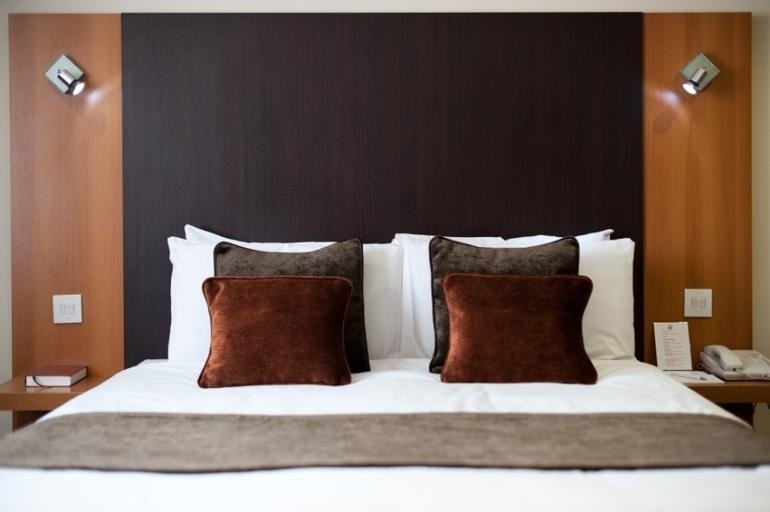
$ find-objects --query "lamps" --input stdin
[678,52,720,97]
[43,53,87,99]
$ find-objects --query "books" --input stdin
[25,363,88,387]
[665,367,724,385]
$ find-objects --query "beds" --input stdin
[1,355,770,512]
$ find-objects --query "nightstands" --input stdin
[662,367,770,405]
[0,373,110,412]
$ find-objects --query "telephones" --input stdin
[699,344,770,380]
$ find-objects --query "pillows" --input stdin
[198,276,353,388]
[440,273,601,384]
[397,228,614,354]
[213,238,372,374]
[165,237,378,361]
[424,237,636,361]
[427,235,581,373]
[181,224,401,357]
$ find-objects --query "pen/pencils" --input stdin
[679,375,706,380]
